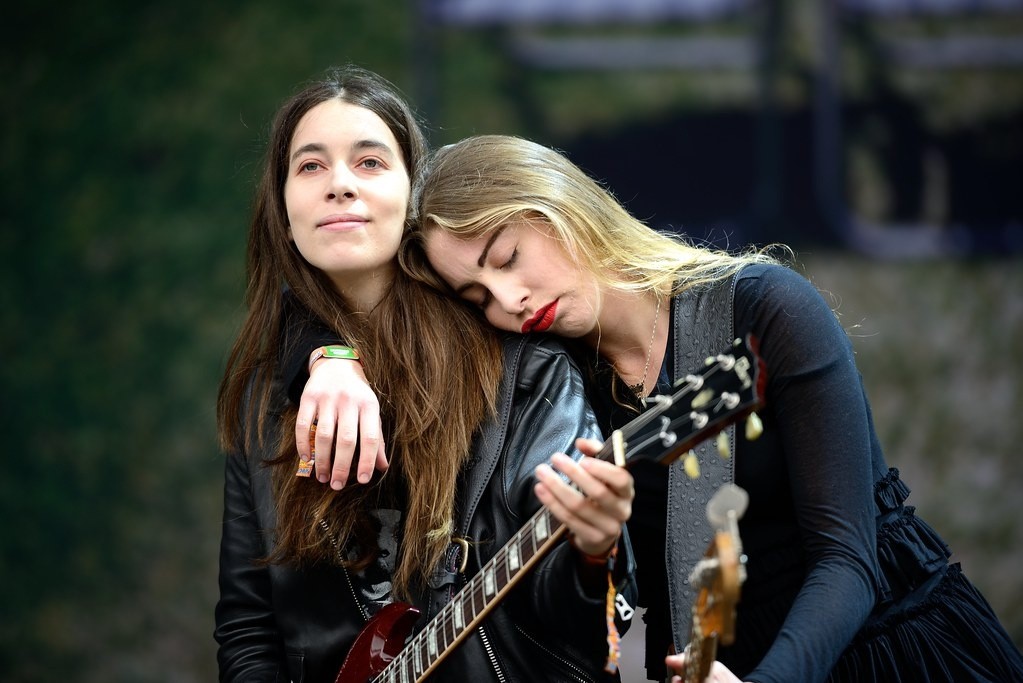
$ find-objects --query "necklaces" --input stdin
[606,296,663,395]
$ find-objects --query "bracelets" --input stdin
[308,345,363,371]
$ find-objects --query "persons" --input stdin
[398,133,1021,683]
[215,66,1022,683]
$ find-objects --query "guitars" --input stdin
[678,480,751,683]
[329,326,768,683]
